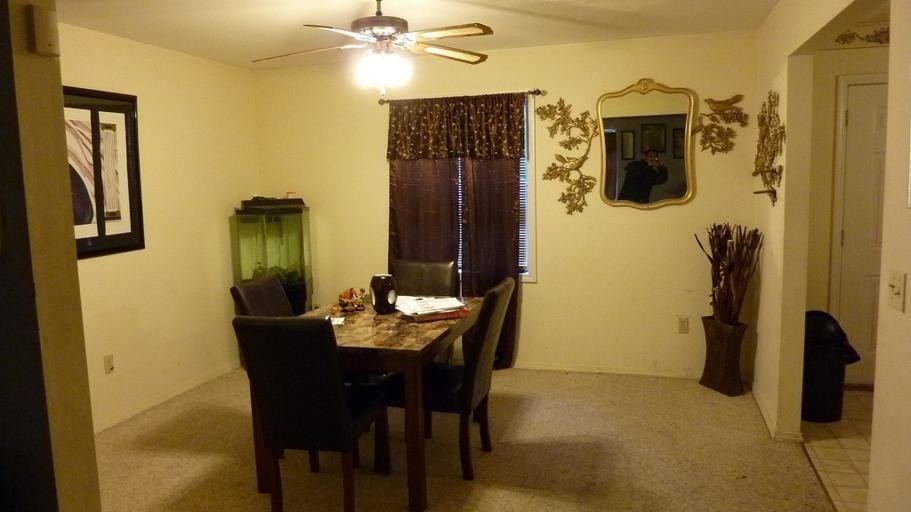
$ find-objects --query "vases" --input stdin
[699,315,748,397]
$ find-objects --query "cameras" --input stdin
[652,154,660,161]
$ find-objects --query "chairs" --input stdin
[229,272,307,459]
[391,259,460,296]
[372,276,516,480]
[231,314,390,512]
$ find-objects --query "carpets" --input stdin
[94,366,835,511]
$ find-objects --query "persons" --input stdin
[617,149,668,203]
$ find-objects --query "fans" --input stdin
[249,0,492,65]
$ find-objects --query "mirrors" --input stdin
[596,78,698,211]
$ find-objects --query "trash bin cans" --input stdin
[802,310,861,423]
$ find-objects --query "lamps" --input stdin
[354,50,414,94]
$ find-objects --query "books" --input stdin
[394,295,470,322]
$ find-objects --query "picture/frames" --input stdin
[620,130,634,160]
[61,84,146,261]
[641,122,666,154]
[673,128,684,159]
